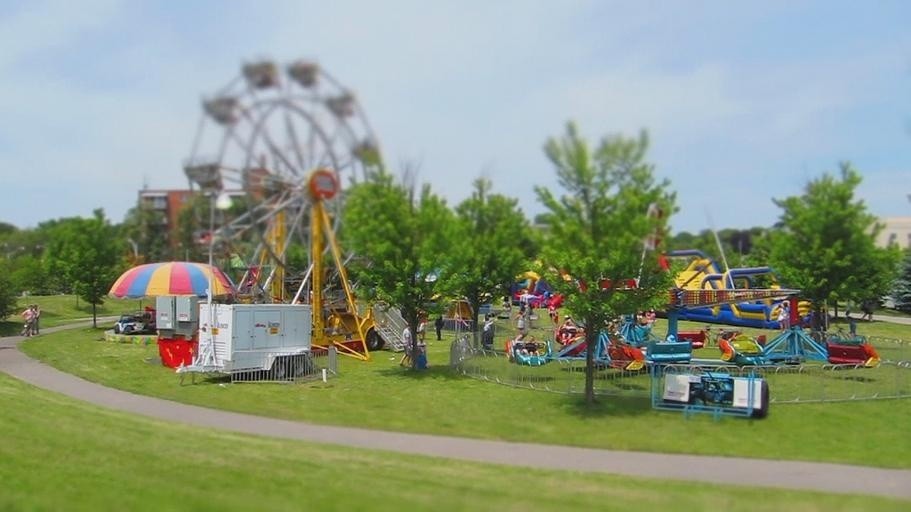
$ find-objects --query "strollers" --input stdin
[21,317,37,336]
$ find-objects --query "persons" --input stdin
[400,295,855,369]
[31,304,40,336]
[21,305,37,335]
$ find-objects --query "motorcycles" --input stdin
[114,314,154,335]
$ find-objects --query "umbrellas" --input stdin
[108,262,238,342]
